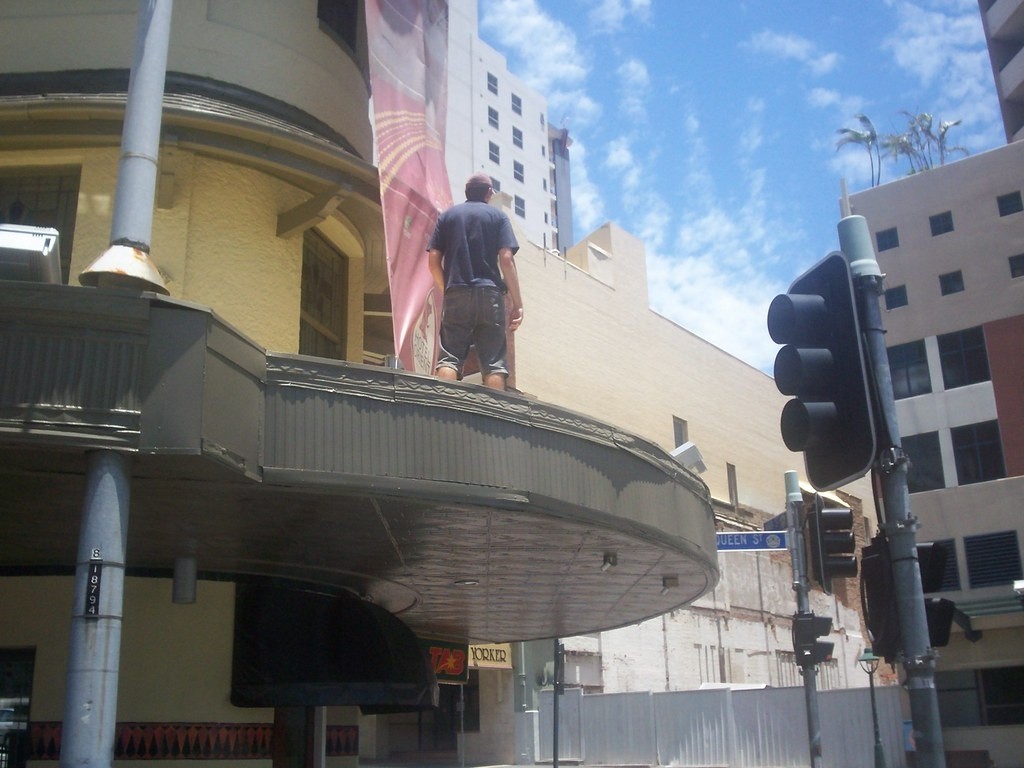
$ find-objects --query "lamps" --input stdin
[601,552,618,571]
[172,555,196,605]
[661,576,680,597]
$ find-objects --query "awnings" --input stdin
[230,575,441,716]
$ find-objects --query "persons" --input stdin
[427,173,524,393]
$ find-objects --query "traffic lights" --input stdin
[915,539,947,576]
[926,599,956,648]
[809,503,858,594]
[810,609,835,665]
[768,250,878,490]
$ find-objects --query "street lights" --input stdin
[858,646,890,768]
